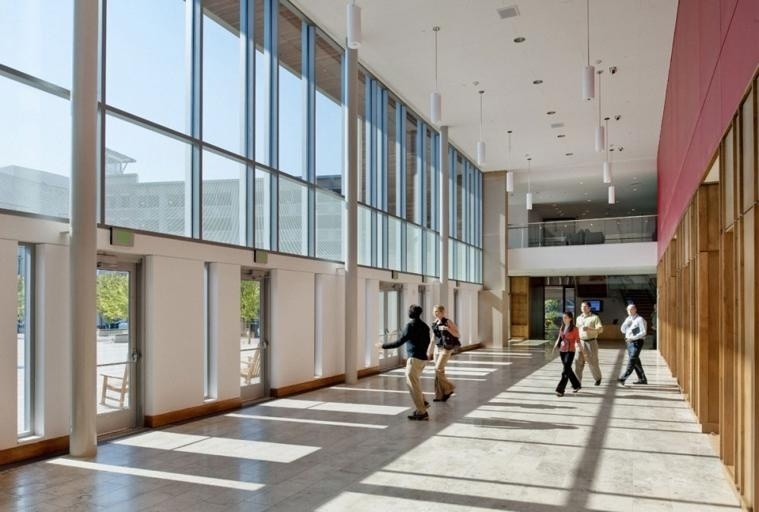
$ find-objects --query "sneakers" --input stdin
[407,401,431,421]
[554,390,565,396]
[572,385,581,393]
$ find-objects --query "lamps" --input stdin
[506,131,514,193]
[526,158,533,210]
[594,70,604,153]
[604,117,611,184]
[346,0,363,49]
[609,149,615,205]
[477,90,486,167]
[582,1,594,101]
[431,27,442,125]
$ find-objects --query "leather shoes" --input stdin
[433,391,454,402]
[617,378,647,386]
[594,378,601,386]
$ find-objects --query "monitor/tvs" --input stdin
[583,300,603,313]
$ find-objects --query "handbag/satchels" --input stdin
[632,327,644,347]
[441,319,461,350]
[557,336,562,348]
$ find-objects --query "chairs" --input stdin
[100,364,127,407]
[240,343,261,385]
[376,334,395,358]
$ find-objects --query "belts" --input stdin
[583,338,597,342]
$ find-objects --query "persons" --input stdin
[615,303,649,386]
[427,303,461,403]
[372,303,431,421]
[572,300,604,391]
[649,303,657,350]
[551,309,592,397]
